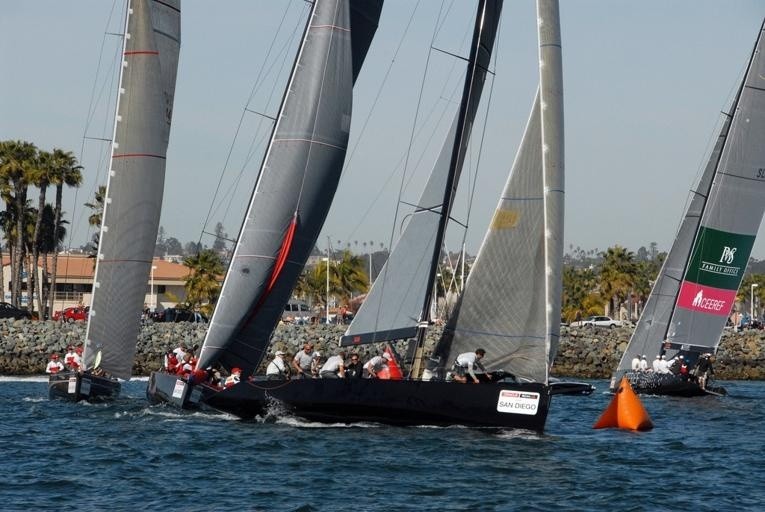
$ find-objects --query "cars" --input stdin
[582,316,622,329]
[0,302,32,320]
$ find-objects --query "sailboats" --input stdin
[609,17,765,396]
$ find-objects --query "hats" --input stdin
[275,351,286,356]
[382,352,393,362]
[47,344,83,359]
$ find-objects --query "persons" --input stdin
[287,302,347,326]
[576,309,583,328]
[163,341,256,391]
[422,368,438,381]
[45,340,84,374]
[727,315,765,333]
[142,301,204,323]
[631,352,716,390]
[266,342,404,380]
[446,349,491,384]
